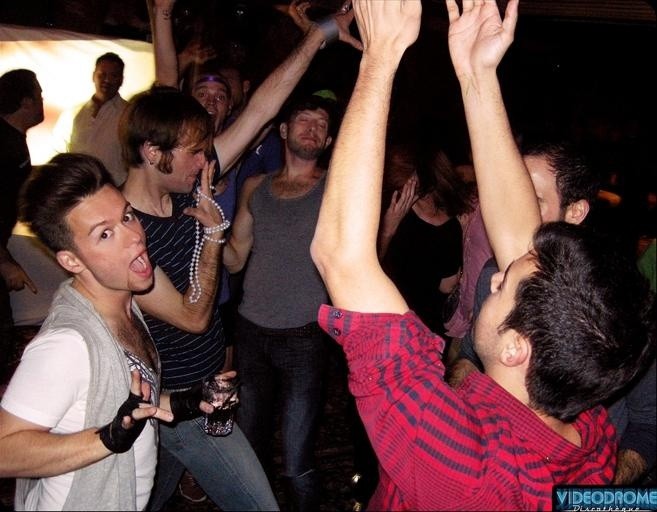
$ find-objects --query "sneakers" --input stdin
[178,470,206,503]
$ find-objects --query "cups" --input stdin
[201,374,242,438]
